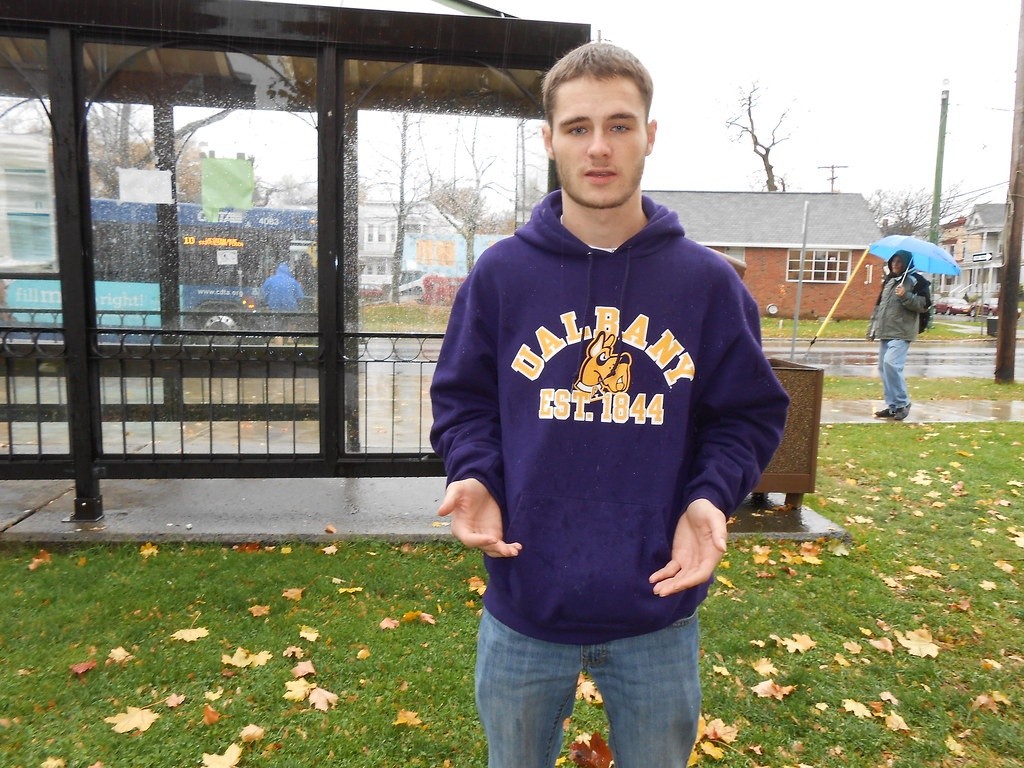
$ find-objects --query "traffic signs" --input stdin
[972,252,992,262]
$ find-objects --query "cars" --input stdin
[934,296,971,315]
[970,298,1021,320]
[357,284,382,297]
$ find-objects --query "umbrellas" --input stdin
[866,234,962,287]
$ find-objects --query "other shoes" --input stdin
[893,402,911,420]
[875,409,896,417]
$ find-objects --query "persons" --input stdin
[427,41,795,768]
[258,262,305,310]
[297,252,319,294]
[865,250,930,421]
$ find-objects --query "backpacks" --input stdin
[910,273,932,334]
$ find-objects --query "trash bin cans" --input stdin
[750,358,825,508]
[986,315,998,335]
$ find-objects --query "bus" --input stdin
[0,193,317,345]
[388,231,515,307]
